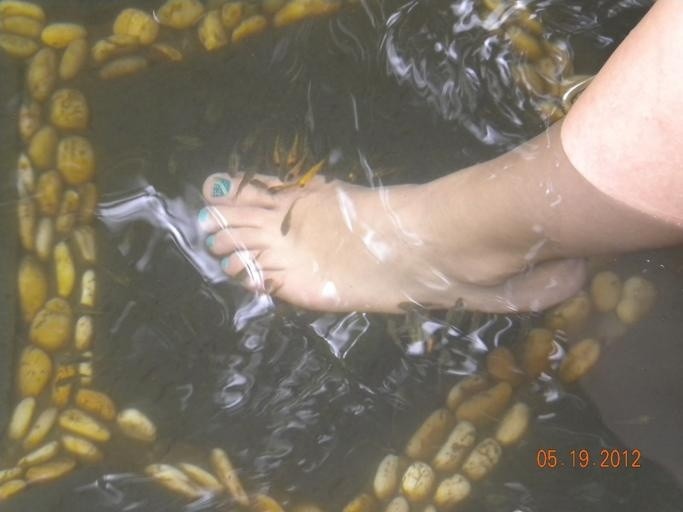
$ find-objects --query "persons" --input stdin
[197,1,682,315]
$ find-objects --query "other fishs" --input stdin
[57,0,682,511]
